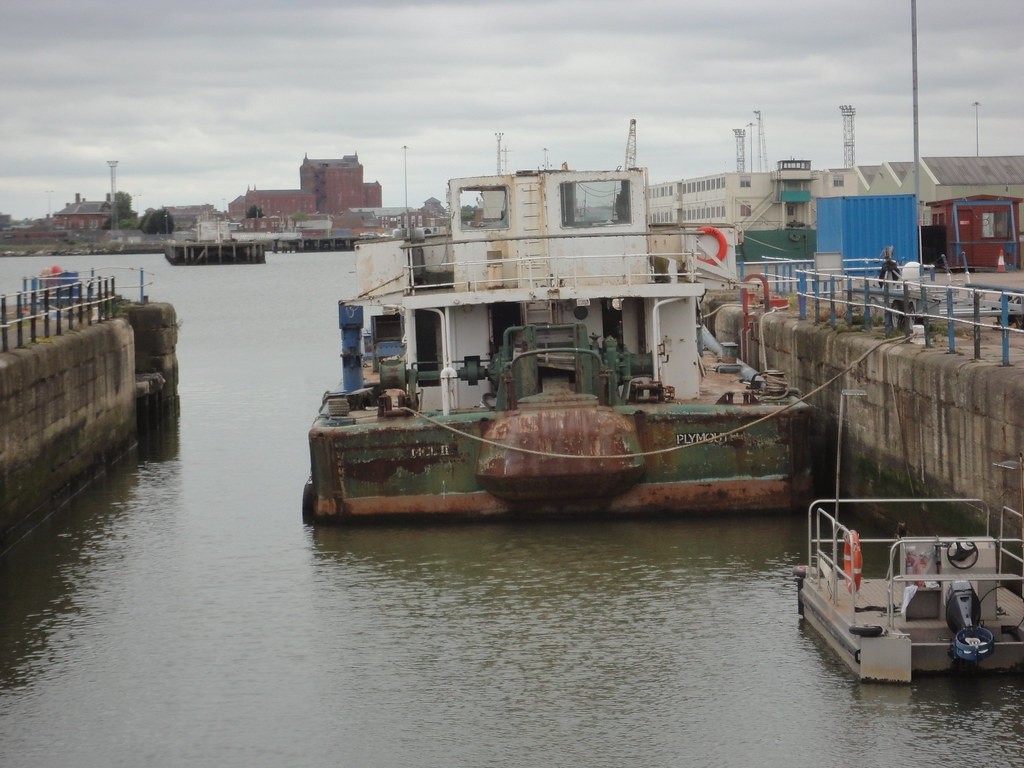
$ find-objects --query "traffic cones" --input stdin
[997,250,1007,274]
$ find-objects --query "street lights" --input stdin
[399,146,411,227]
[971,102,982,156]
[747,123,758,173]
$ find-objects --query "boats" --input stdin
[794,497,1024,683]
[302,165,820,526]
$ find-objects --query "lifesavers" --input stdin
[693,226,728,266]
[843,530,863,593]
